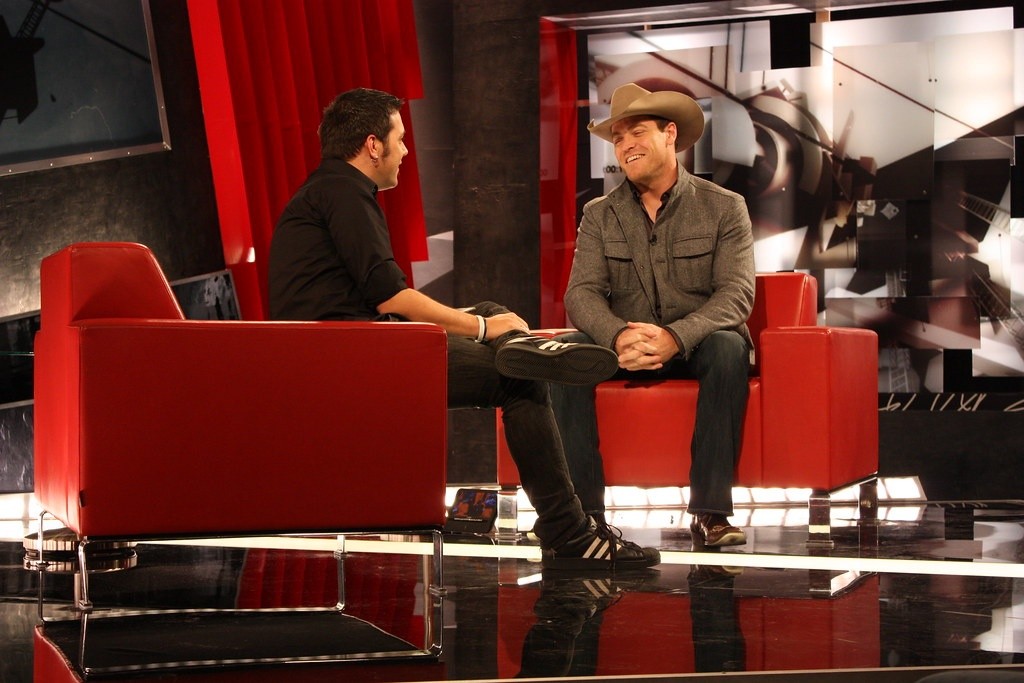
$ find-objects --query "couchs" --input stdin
[32,241,448,675]
[496,273,878,600]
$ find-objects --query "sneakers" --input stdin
[537,567,663,619]
[527,528,541,542]
[494,335,620,389]
[539,517,662,570]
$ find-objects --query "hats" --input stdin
[585,84,706,153]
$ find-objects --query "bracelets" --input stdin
[475,315,487,343]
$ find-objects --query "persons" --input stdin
[546,82,756,546]
[267,88,662,570]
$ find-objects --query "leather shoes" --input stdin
[689,563,746,585]
[686,513,750,548]
